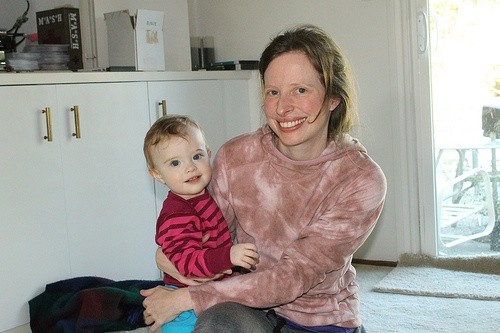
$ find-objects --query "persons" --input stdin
[143,112,259,332]
[140,24,387,333]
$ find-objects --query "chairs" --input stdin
[435,140,496,247]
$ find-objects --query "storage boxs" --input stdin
[37,8,83,72]
[104,7,165,71]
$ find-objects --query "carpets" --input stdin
[373,252,500,300]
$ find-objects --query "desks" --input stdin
[445,143,500,251]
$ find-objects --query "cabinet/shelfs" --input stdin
[0,70,266,332]
